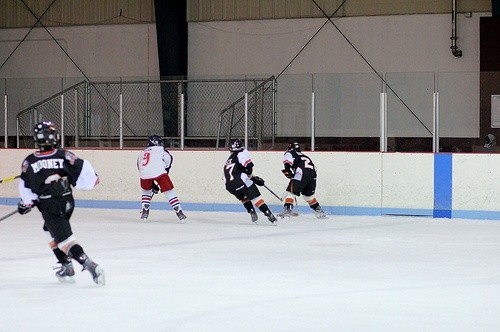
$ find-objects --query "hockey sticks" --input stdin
[290,177,298,208]
[140,184,159,213]
[0,208,18,222]
[263,184,289,201]
[0,173,22,184]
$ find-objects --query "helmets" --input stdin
[148,134,161,146]
[32,121,57,145]
[288,140,301,152]
[230,138,244,151]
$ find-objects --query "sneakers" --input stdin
[264,209,277,225]
[251,211,258,225]
[140,208,149,221]
[81,258,105,286]
[176,210,188,223]
[283,204,293,220]
[56,261,76,281]
[315,206,329,219]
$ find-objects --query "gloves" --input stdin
[251,176,264,186]
[152,184,159,194]
[282,170,294,178]
[18,201,31,214]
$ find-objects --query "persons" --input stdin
[137,135,189,223]
[276,141,329,220]
[17,121,105,284]
[223,138,279,225]
